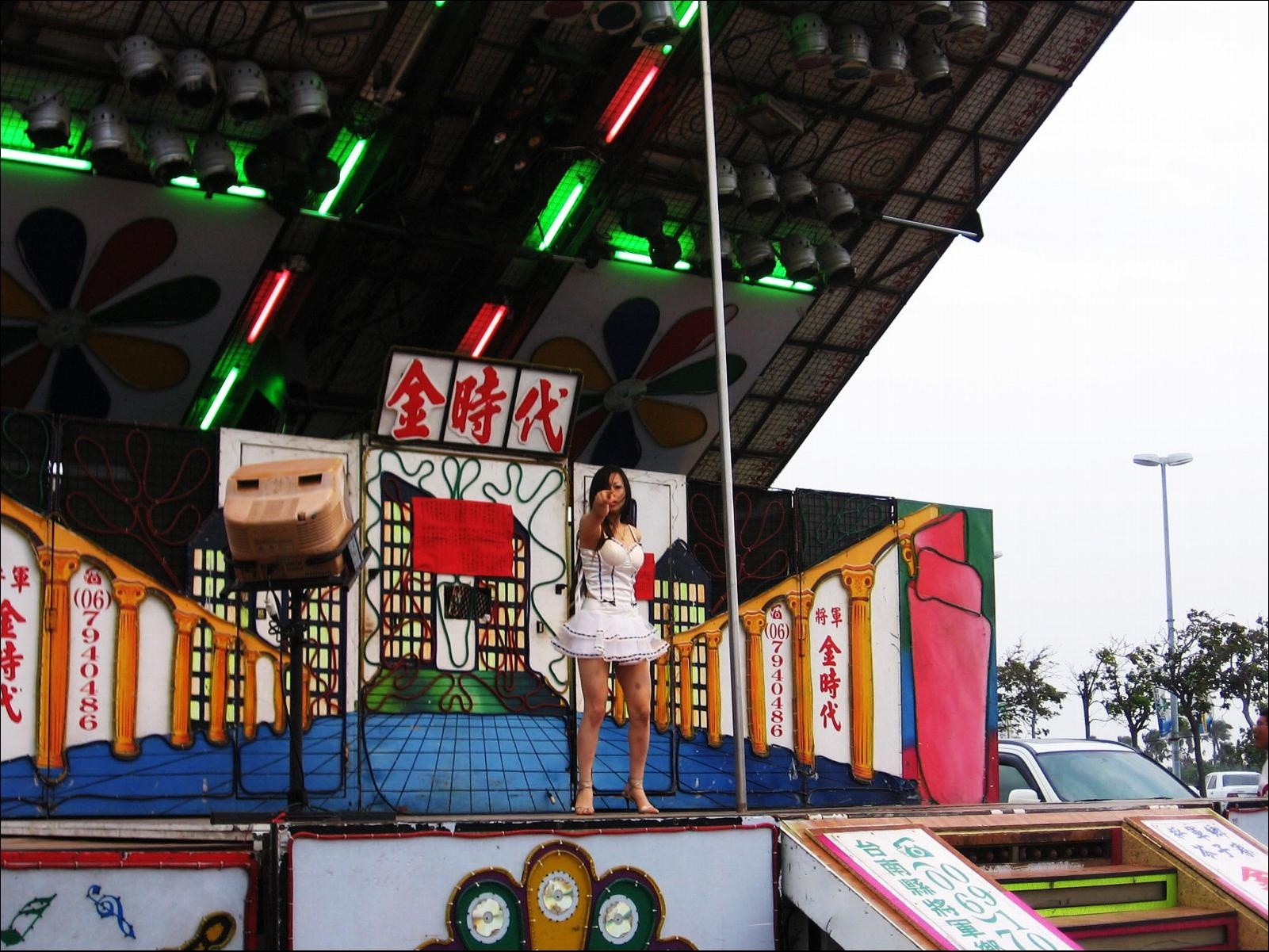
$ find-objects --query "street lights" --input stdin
[1132,452,1193,783]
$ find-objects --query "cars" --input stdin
[990,738,1210,813]
[1204,771,1264,815]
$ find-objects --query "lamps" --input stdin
[695,228,732,276]
[779,171,817,216]
[199,363,241,431]
[739,90,807,144]
[703,158,742,209]
[292,0,391,40]
[170,47,218,105]
[22,86,72,150]
[538,178,584,250]
[738,165,780,211]
[86,98,131,165]
[530,0,685,47]
[195,130,237,189]
[144,119,193,182]
[285,70,331,127]
[781,233,821,282]
[819,183,856,217]
[734,232,777,280]
[117,33,170,99]
[648,231,681,270]
[225,62,273,122]
[788,0,989,94]
[814,239,858,286]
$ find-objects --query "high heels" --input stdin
[622,778,659,815]
[575,781,595,815]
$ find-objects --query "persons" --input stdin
[551,466,671,815]
[1252,706,1269,797]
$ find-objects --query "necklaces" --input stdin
[617,525,626,541]
